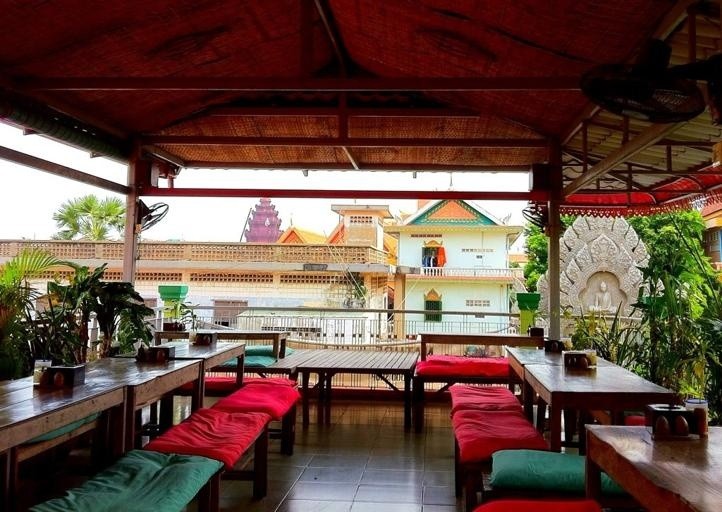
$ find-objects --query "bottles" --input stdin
[38,371,65,388]
[568,357,588,369]
[654,415,688,436]
[138,344,144,362]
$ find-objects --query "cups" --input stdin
[687,398,711,435]
[34,361,52,382]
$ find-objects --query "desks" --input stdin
[296,348,419,433]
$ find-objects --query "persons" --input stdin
[586,281,619,316]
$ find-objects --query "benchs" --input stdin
[416,334,722,511]
[0,330,301,512]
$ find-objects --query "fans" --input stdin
[133,199,170,232]
